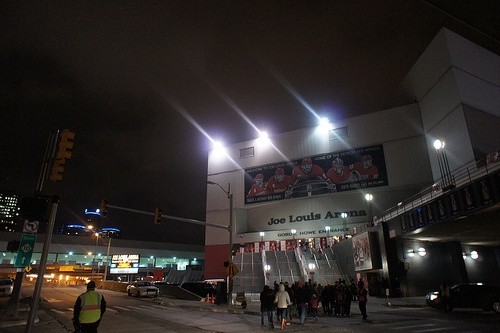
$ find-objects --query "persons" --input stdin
[305,239,338,260]
[373,277,400,298]
[260,277,367,329]
[248,174,268,196]
[440,280,452,316]
[292,158,327,183]
[71,281,106,333]
[265,168,295,192]
[10,276,14,281]
[204,281,215,286]
[348,155,378,178]
[339,236,348,241]
[266,271,272,279]
[326,158,351,183]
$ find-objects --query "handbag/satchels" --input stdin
[358,296,365,301]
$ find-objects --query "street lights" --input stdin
[207,179,236,313]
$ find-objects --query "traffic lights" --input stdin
[50,159,67,184]
[100,199,108,216]
[56,128,77,160]
[154,207,161,225]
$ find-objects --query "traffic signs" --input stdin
[14,233,36,270]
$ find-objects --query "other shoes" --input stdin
[362,318,366,320]
[261,323,264,325]
[315,318,318,320]
[270,326,274,328]
[301,323,304,327]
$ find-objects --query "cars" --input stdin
[0,279,16,296]
[126,280,160,297]
[425,283,500,314]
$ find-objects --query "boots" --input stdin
[280,319,287,330]
